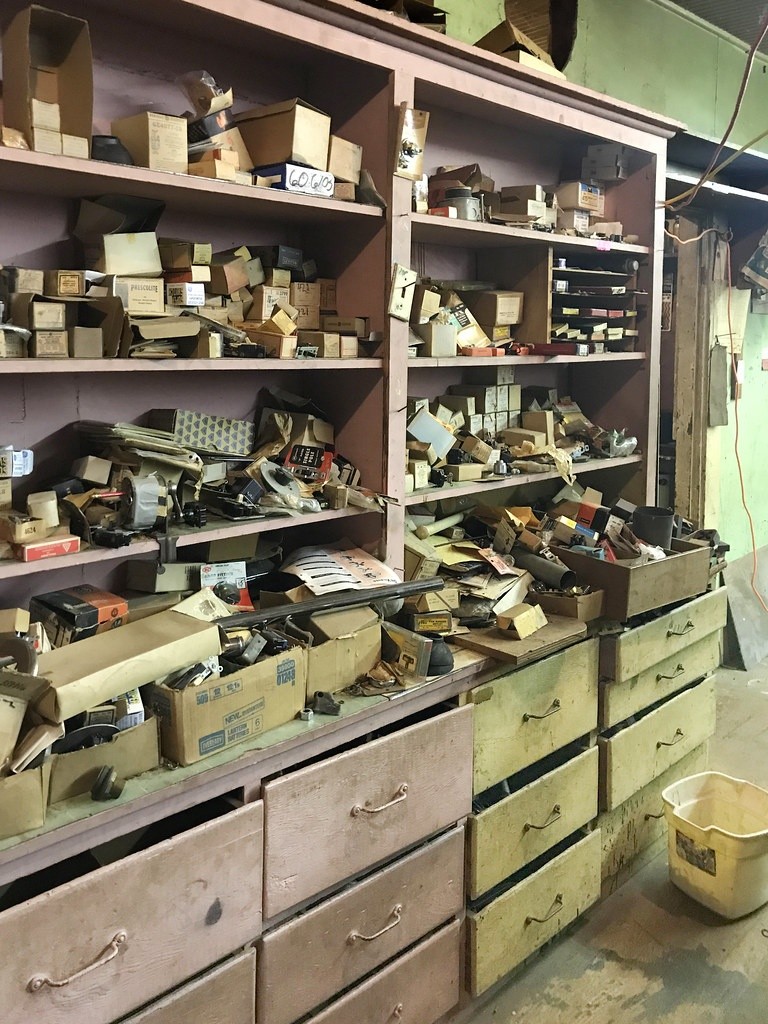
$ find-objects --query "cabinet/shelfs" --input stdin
[2,0,686,608]
[459,584,726,996]
[2,586,474,1023]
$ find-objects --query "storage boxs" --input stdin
[661,771,768,920]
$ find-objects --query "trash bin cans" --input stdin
[659,771,768,920]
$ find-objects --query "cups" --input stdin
[632,506,674,551]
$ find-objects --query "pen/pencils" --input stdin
[88,492,125,498]
